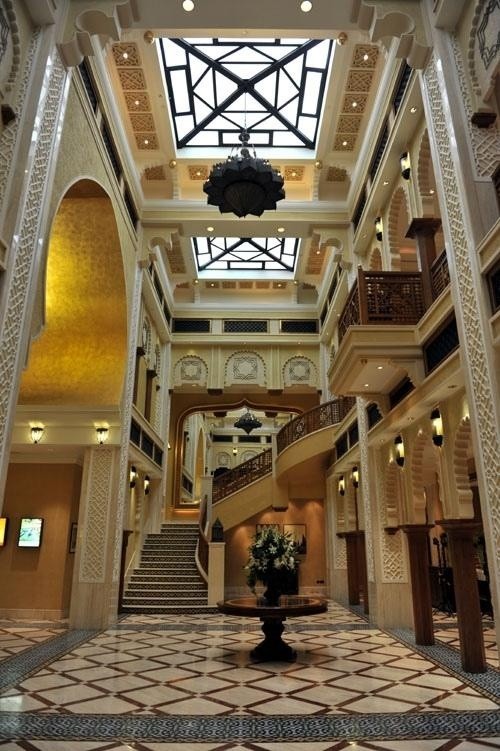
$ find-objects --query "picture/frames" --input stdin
[68,523,79,554]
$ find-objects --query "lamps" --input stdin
[203,80,288,222]
[233,409,262,437]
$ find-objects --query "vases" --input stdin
[262,578,281,602]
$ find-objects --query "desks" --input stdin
[217,596,329,665]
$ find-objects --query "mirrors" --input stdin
[165,384,321,522]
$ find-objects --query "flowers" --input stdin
[241,528,302,598]
